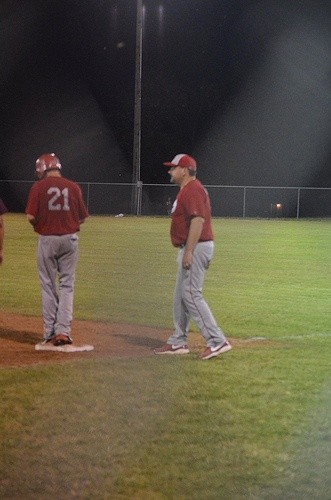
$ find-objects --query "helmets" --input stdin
[35,153,61,177]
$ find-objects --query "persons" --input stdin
[0,199,9,264]
[154,153,232,359]
[25,153,88,346]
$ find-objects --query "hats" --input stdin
[163,154,197,170]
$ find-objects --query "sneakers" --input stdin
[155,344,189,355]
[55,334,71,346]
[35,337,57,348]
[203,341,231,359]
